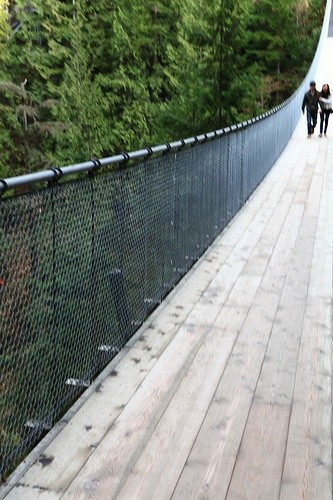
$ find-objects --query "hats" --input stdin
[309,81,316,85]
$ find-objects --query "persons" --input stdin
[302,81,325,139]
[318,84,332,138]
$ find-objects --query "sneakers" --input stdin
[307,134,311,139]
[324,134,328,137]
[317,132,322,137]
[311,125,314,133]
